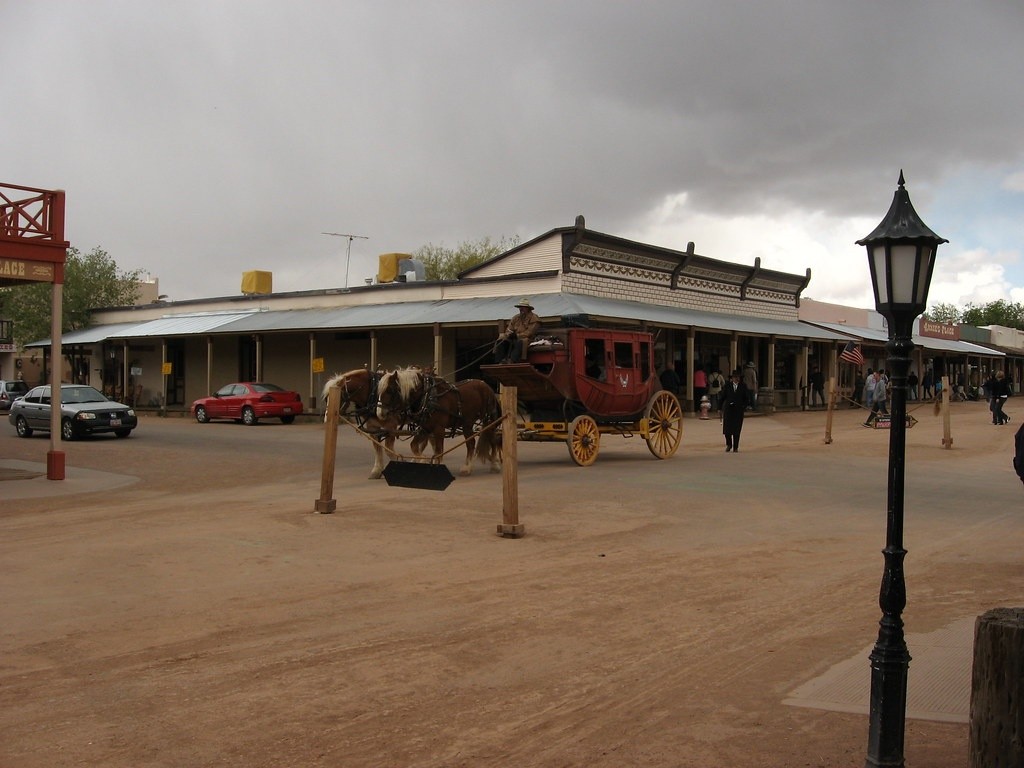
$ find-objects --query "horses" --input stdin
[375,368,502,475]
[322,369,429,479]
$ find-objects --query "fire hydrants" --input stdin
[699,395,712,420]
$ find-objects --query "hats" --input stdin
[728,370,745,379]
[514,298,535,310]
[747,362,757,368]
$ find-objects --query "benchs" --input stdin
[508,337,563,365]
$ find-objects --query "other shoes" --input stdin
[726,444,732,451]
[733,448,738,452]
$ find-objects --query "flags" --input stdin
[840,340,863,365]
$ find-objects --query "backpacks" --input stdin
[712,374,720,388]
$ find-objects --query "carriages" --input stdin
[320,327,684,480]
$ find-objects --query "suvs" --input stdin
[0,379,32,411]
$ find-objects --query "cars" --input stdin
[190,381,304,426]
[8,384,138,442]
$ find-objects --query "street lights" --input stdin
[853,167,952,768]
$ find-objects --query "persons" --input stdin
[1013,422,1024,484]
[921,372,933,401]
[980,369,1011,425]
[799,367,825,407]
[16,369,23,380]
[694,362,758,452]
[494,299,541,363]
[908,372,918,400]
[950,383,964,402]
[659,362,681,418]
[850,368,889,428]
[584,354,601,379]
